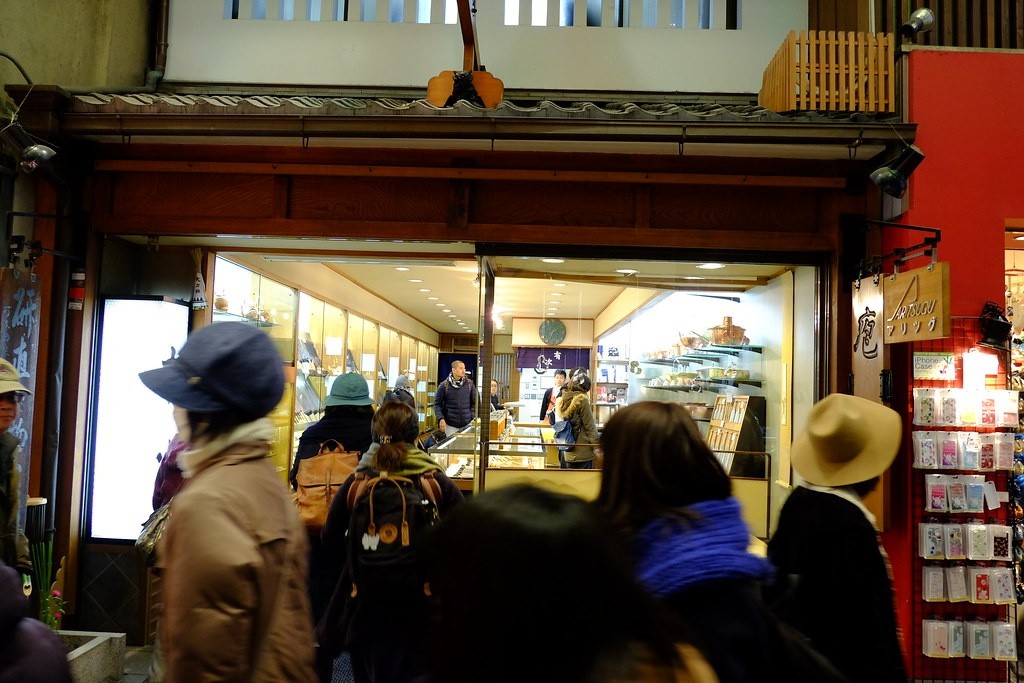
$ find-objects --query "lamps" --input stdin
[902,8,936,45]
[869,144,925,200]
[0,117,58,182]
[975,300,1012,353]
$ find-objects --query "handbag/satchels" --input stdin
[134,503,173,562]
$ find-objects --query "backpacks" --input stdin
[552,413,584,452]
[345,469,443,599]
[293,439,361,534]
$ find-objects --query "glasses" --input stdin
[0,392,24,403]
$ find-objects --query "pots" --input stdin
[649,316,750,387]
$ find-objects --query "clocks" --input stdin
[539,319,566,346]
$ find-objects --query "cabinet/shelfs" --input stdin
[211,251,439,496]
[639,343,763,423]
[590,358,629,432]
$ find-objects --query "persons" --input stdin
[291,371,375,683]
[436,361,480,437]
[408,484,715,683]
[394,374,414,408]
[550,367,602,469]
[539,370,567,419]
[0,357,77,683]
[586,400,774,683]
[323,402,464,683]
[766,390,911,683]
[491,380,504,411]
[139,321,315,683]
[143,433,194,683]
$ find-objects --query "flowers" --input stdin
[39,589,68,633]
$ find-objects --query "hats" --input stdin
[0,358,31,395]
[395,376,410,387]
[789,392,903,487]
[138,320,284,423]
[324,371,375,407]
